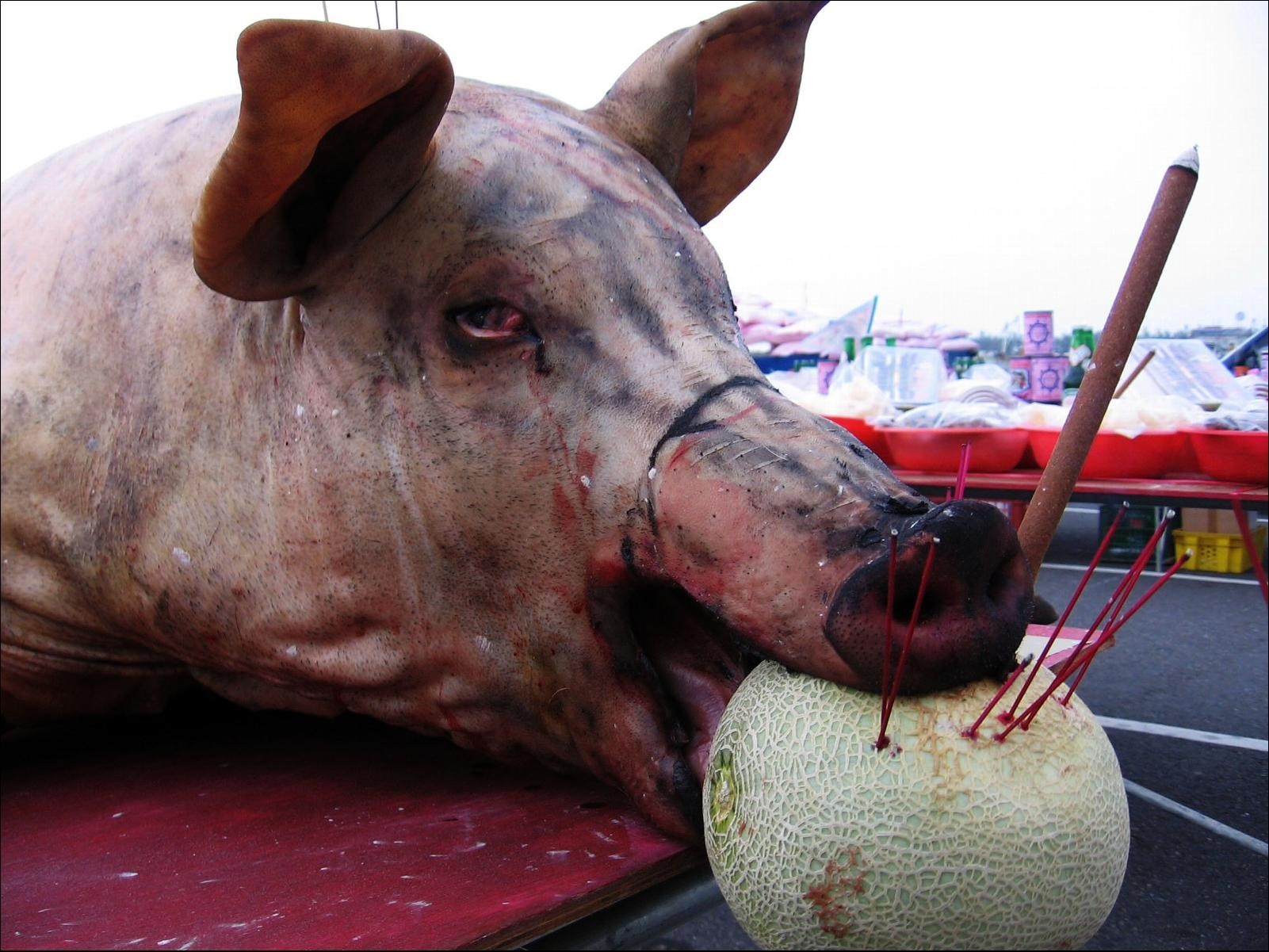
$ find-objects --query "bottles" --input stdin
[1061,326,1097,405]
[843,334,896,366]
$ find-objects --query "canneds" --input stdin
[1007,310,1064,405]
[952,355,982,374]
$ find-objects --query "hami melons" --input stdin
[702,649,1130,951]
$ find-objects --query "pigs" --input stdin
[1,0,1025,848]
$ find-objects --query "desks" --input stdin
[0,625,1115,952]
[891,459,1269,615]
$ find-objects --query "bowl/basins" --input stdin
[820,415,1268,481]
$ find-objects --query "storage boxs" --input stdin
[1182,506,1258,535]
[1047,503,1120,555]
[1172,527,1266,573]
